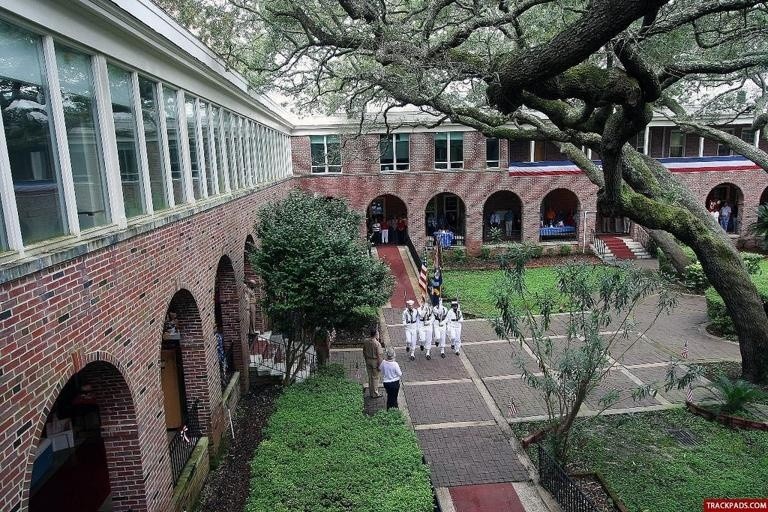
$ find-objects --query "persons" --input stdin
[433,297,448,358]
[707,195,737,234]
[448,301,463,356]
[621,214,631,236]
[212,321,224,377]
[415,296,436,358]
[544,207,556,226]
[427,214,464,249]
[401,298,418,360]
[599,211,613,234]
[363,329,384,400]
[556,209,566,226]
[315,324,330,367]
[504,206,514,237]
[367,214,407,244]
[378,347,404,412]
[490,207,502,238]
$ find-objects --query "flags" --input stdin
[418,248,428,292]
[431,241,442,306]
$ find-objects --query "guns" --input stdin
[404,290,409,324]
[456,288,460,320]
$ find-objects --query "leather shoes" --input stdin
[403,340,463,360]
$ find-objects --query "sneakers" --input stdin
[369,393,383,398]
